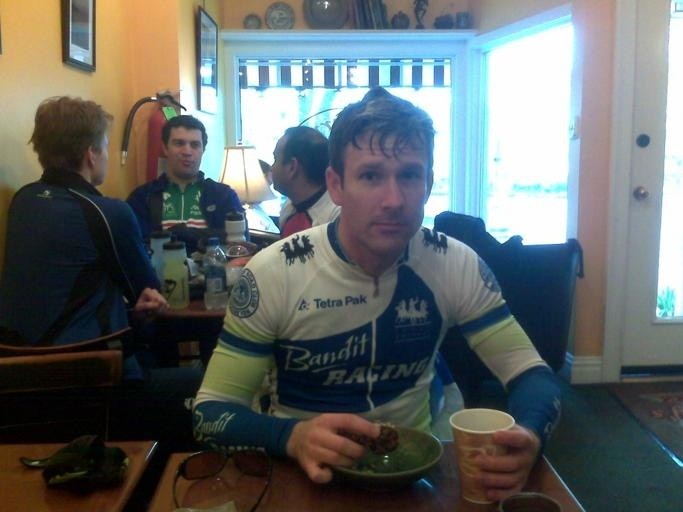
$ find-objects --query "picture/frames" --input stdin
[57,0,100,74]
[193,5,220,118]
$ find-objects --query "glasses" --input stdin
[172,443,274,512]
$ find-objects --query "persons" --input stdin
[2,93,197,439]
[268,125,341,240]
[190,85,562,499]
[243,158,280,235]
[124,113,250,249]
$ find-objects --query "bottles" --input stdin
[149,205,253,312]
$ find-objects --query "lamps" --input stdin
[213,144,277,231]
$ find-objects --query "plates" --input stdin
[303,0,354,30]
[265,2,295,31]
[243,15,261,28]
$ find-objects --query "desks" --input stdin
[0,437,159,509]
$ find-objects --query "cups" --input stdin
[453,12,473,30]
[499,492,565,512]
[448,408,516,506]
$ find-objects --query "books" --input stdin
[348,1,388,30]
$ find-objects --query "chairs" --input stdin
[448,237,582,416]
[0,319,135,381]
[0,346,125,439]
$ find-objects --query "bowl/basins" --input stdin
[323,423,444,489]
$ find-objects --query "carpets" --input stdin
[539,384,683,510]
[600,378,682,471]
